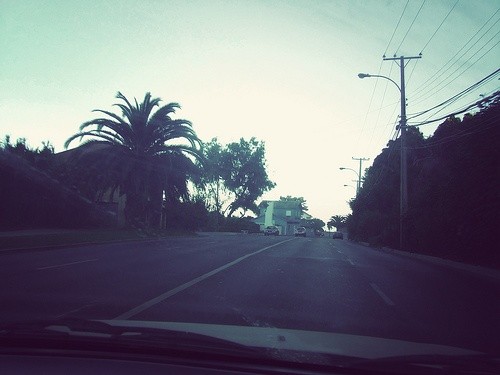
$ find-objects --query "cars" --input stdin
[332,231,344,240]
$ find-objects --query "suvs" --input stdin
[294,226,307,236]
[263,226,279,236]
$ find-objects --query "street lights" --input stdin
[339,156,371,198]
[357,55,424,251]
